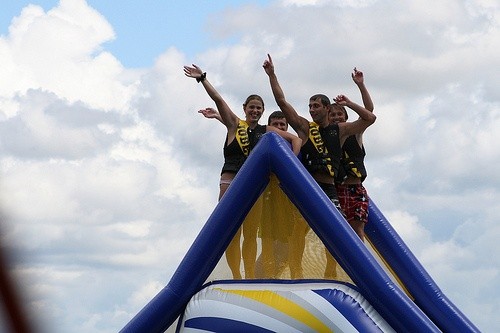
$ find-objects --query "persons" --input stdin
[262,52,377,279]
[198,108,293,279]
[183,63,304,280]
[328,68,374,244]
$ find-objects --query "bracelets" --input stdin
[196,72,207,83]
